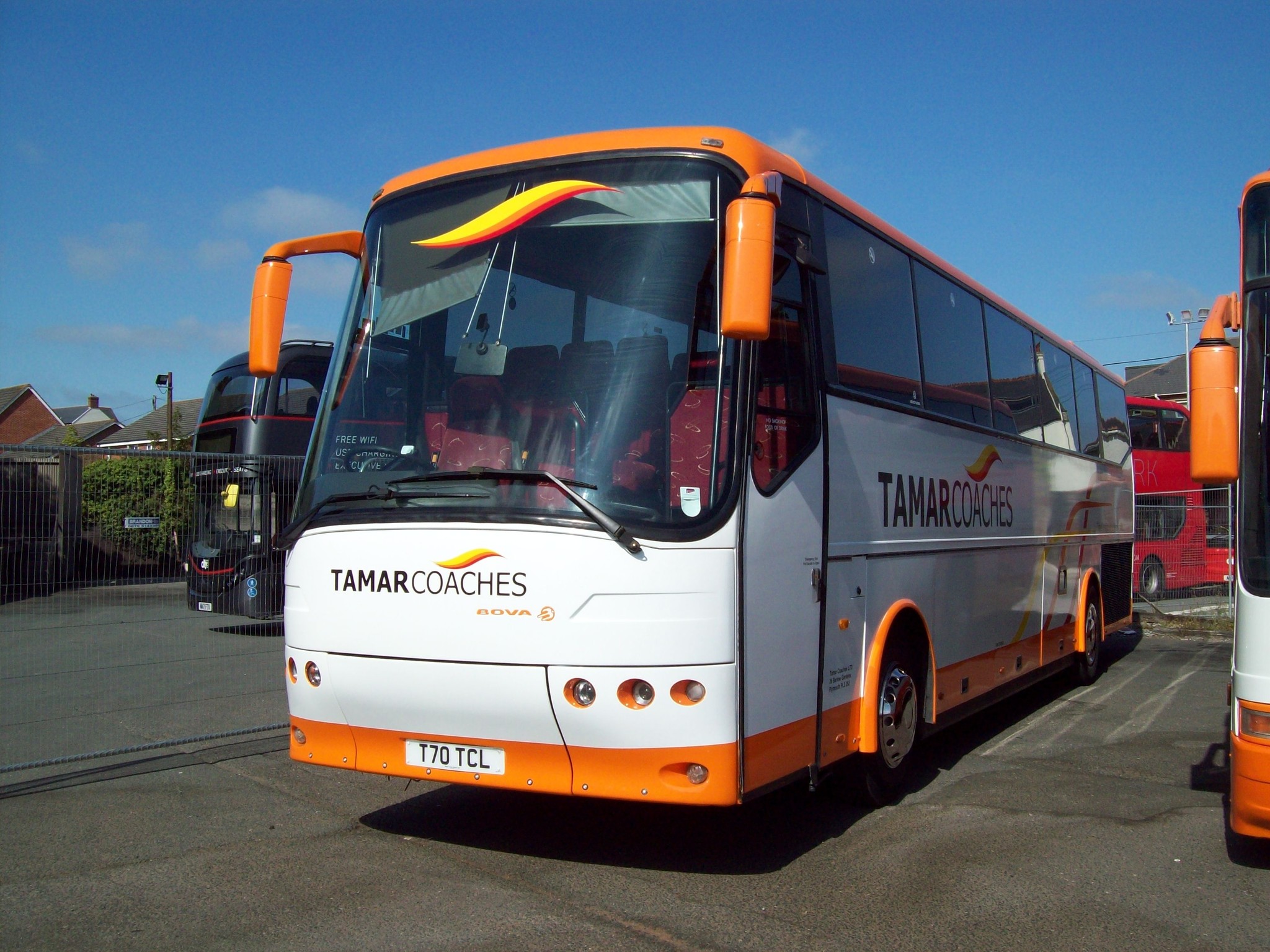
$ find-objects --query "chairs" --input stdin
[421,335,672,509]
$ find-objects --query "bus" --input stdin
[1123,395,1236,602]
[249,125,1138,818]
[185,337,339,620]
[1192,168,1270,840]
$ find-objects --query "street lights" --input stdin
[157,372,174,451]
